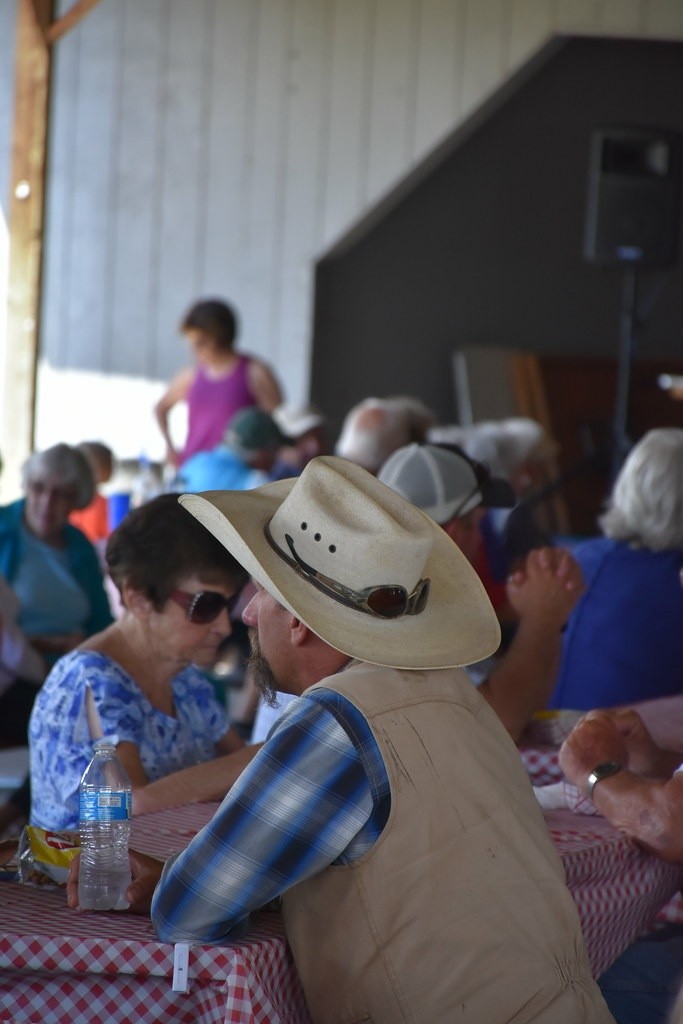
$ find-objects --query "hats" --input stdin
[177,456,502,671]
[377,443,484,523]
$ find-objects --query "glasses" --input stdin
[284,536,432,620]
[149,582,239,623]
[440,443,488,525]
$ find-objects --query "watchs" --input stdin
[587,760,621,800]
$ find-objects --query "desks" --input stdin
[0,747,683,1024]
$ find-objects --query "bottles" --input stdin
[77,741,133,910]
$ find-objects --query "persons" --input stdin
[547,429,683,711]
[0,441,161,750]
[178,394,586,750]
[558,705,683,861]
[24,491,275,833]
[150,297,284,467]
[69,456,617,1024]
